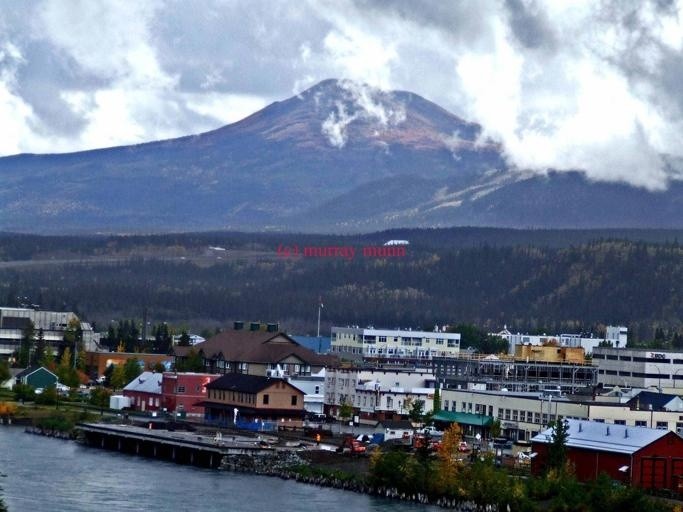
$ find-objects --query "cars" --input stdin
[373,425,519,456]
